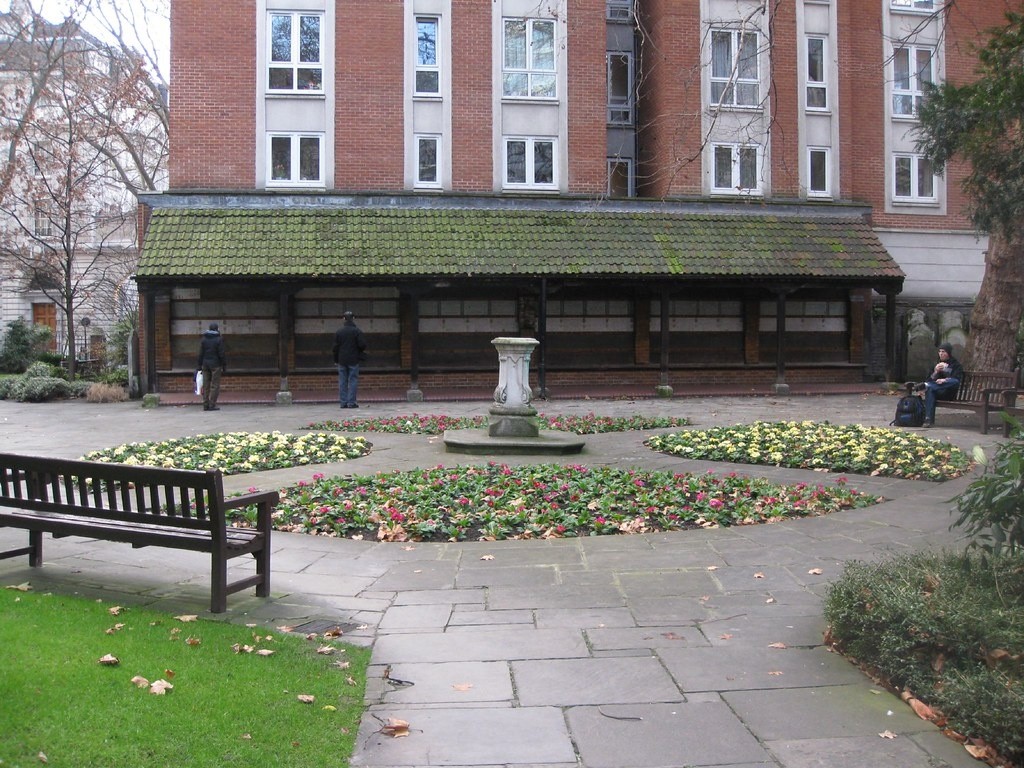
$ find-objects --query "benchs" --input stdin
[0,452,280,613]
[59,359,100,384]
[903,368,1020,434]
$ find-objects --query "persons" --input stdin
[332,311,366,408]
[197,323,226,411]
[912,343,963,428]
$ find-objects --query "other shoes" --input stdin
[204,407,220,411]
[349,404,359,408]
[914,382,925,392]
[340,404,348,408]
[922,421,935,428]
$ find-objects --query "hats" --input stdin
[939,343,952,353]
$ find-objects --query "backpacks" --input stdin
[890,395,924,427]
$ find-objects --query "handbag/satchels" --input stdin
[194,371,204,396]
